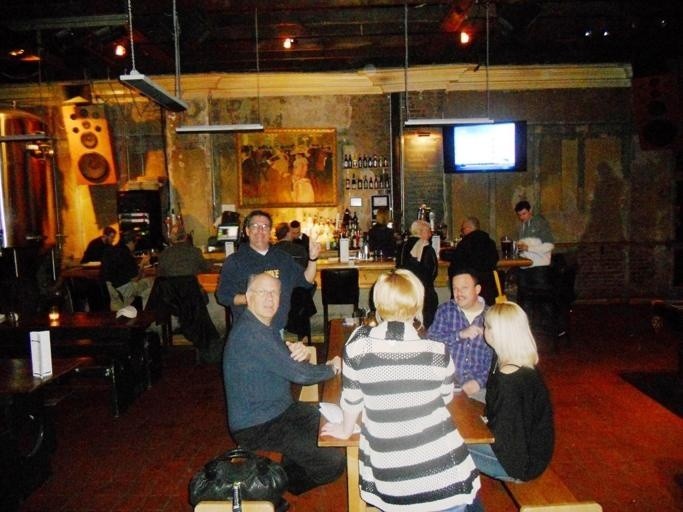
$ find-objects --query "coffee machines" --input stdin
[436,222,448,248]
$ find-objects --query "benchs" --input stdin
[196,267,605,512]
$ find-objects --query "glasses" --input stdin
[247,222,271,232]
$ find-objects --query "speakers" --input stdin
[61,103,118,186]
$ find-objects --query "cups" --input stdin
[339,239,348,263]
[225,242,233,256]
[46,303,59,320]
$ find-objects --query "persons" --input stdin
[241,144,333,203]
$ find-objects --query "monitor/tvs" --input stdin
[443,120,527,173]
[215,224,241,244]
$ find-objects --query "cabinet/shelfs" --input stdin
[340,165,389,197]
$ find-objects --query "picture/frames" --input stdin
[234,127,338,207]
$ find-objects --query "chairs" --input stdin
[0,261,208,511]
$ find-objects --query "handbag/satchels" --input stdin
[187,446,291,510]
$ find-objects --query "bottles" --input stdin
[356,244,383,259]
[342,153,389,168]
[448,235,462,248]
[302,208,363,250]
[345,174,389,189]
[500,233,512,253]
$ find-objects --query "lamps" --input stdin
[173,1,264,136]
[399,0,498,129]
[1,29,53,143]
[117,0,190,114]
